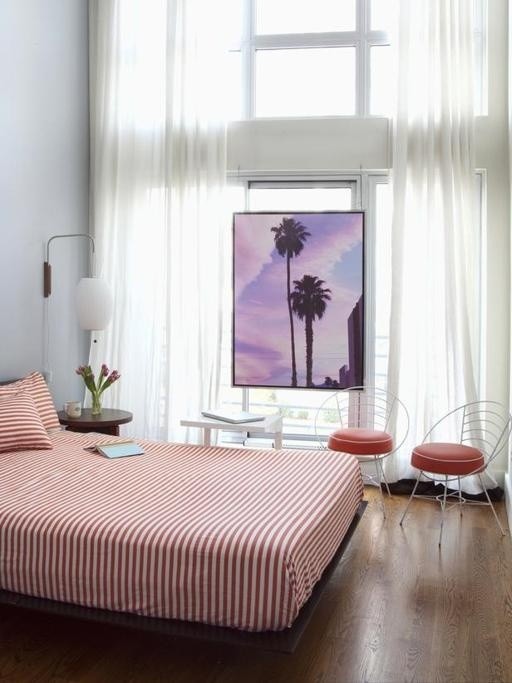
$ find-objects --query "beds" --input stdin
[0,429,365,656]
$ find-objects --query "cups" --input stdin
[64,400,82,419]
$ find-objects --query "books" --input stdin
[201,406,266,425]
[85,440,145,459]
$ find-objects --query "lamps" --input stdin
[44,232,112,385]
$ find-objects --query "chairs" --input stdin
[313,385,412,521]
[400,399,511,547]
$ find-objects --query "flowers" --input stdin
[74,365,121,414]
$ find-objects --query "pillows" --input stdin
[0,392,53,452]
[0,369,64,432]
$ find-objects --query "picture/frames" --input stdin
[231,208,368,392]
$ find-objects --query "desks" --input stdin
[55,408,133,436]
[180,407,284,449]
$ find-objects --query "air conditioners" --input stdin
[224,115,394,186]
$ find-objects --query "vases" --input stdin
[91,391,104,414]
